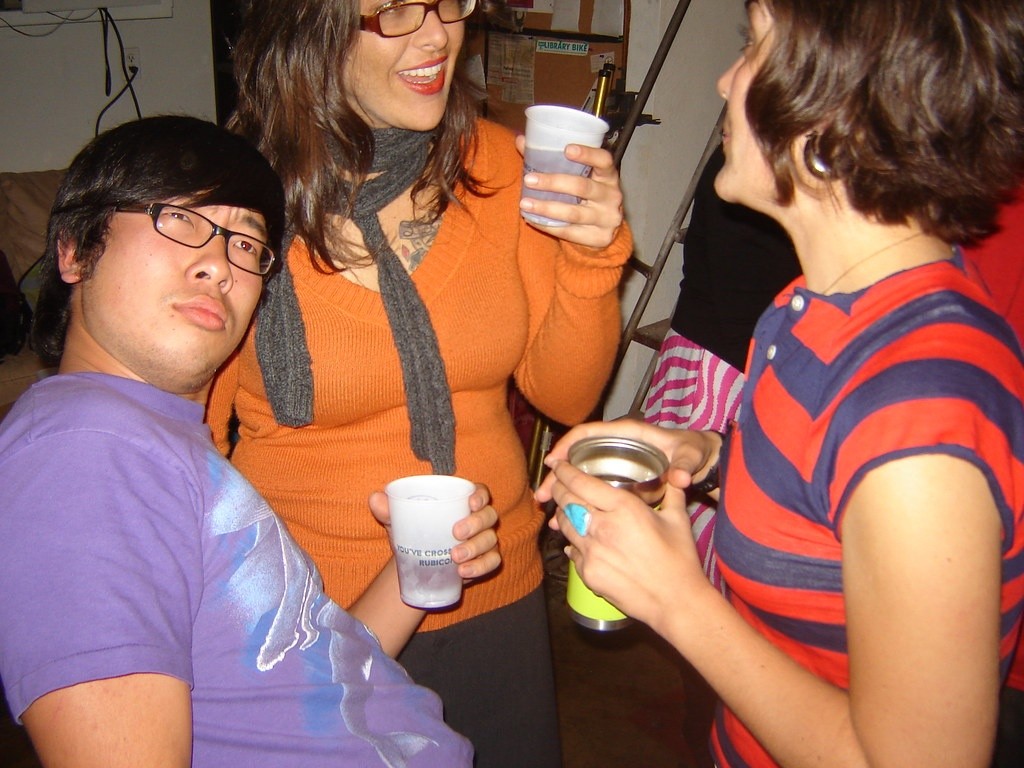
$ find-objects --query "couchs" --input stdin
[0,167,72,409]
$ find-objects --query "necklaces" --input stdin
[822,231,924,294]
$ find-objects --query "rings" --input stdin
[563,502,596,537]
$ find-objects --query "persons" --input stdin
[182,0,632,768]
[645,143,803,604]
[533,1,1024,768]
[0,116,502,768]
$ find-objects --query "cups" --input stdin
[384,475,477,607]
[519,104,610,229]
[563,438,670,633]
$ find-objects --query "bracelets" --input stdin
[687,429,727,495]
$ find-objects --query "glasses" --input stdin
[108,202,276,282]
[360,0,476,38]
